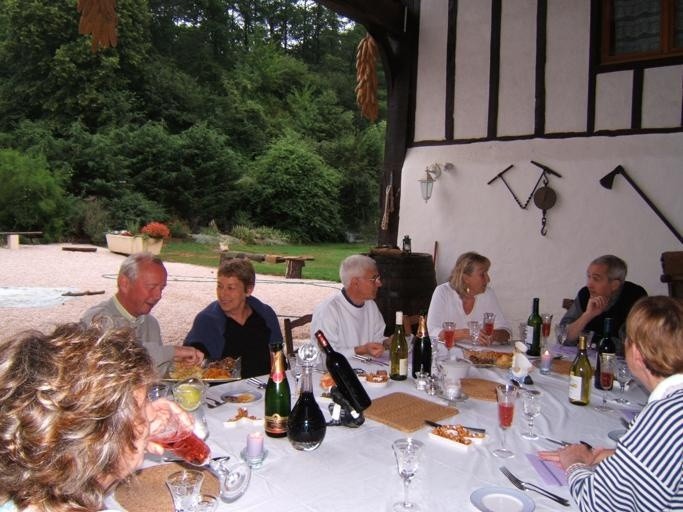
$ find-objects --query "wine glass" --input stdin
[493,382,515,460]
[391,437,425,512]
[517,391,542,441]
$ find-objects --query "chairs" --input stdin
[402,312,427,335]
[284,312,312,370]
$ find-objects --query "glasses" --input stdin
[358,274,381,285]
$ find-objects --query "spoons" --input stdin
[423,419,485,433]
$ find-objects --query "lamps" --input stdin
[415,161,441,205]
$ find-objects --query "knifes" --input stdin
[545,437,564,446]
[246,376,267,390]
[352,353,390,368]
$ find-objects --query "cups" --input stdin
[143,377,250,512]
[286,352,302,380]
[414,312,507,401]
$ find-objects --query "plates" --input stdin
[220,388,263,405]
[608,429,627,442]
[469,486,536,512]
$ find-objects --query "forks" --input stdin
[500,467,571,507]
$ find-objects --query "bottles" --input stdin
[314,329,372,414]
[245,429,266,470]
[287,361,326,452]
[523,297,633,413]
[403,235,411,254]
[264,342,292,438]
[389,311,409,381]
[411,314,432,378]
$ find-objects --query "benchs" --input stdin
[215,249,314,279]
[0,231,43,244]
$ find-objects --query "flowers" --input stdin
[140,222,170,238]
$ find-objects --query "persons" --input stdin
[184,259,287,387]
[310,254,395,359]
[80,252,204,402]
[538,296,682,512]
[0,317,194,511]
[557,255,648,358]
[426,252,512,347]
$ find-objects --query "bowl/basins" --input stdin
[366,379,388,389]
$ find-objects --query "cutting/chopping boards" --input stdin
[360,391,460,434]
